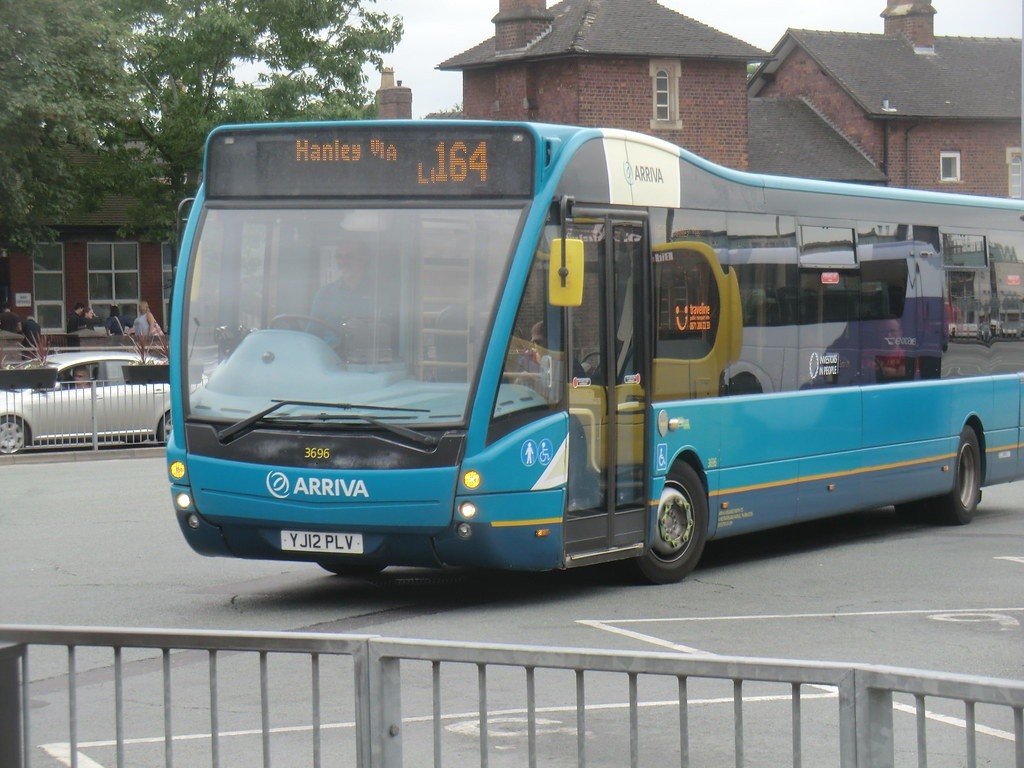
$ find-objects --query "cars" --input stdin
[1,353,208,455]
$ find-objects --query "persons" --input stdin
[58,368,90,389]
[0,303,22,333]
[104,306,135,336]
[18,315,41,359]
[514,322,587,393]
[66,303,99,333]
[861,314,919,386]
[134,301,165,336]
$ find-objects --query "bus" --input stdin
[164,119,1024,588]
[502,237,744,510]
[713,238,949,395]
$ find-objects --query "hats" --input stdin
[531,320,547,342]
